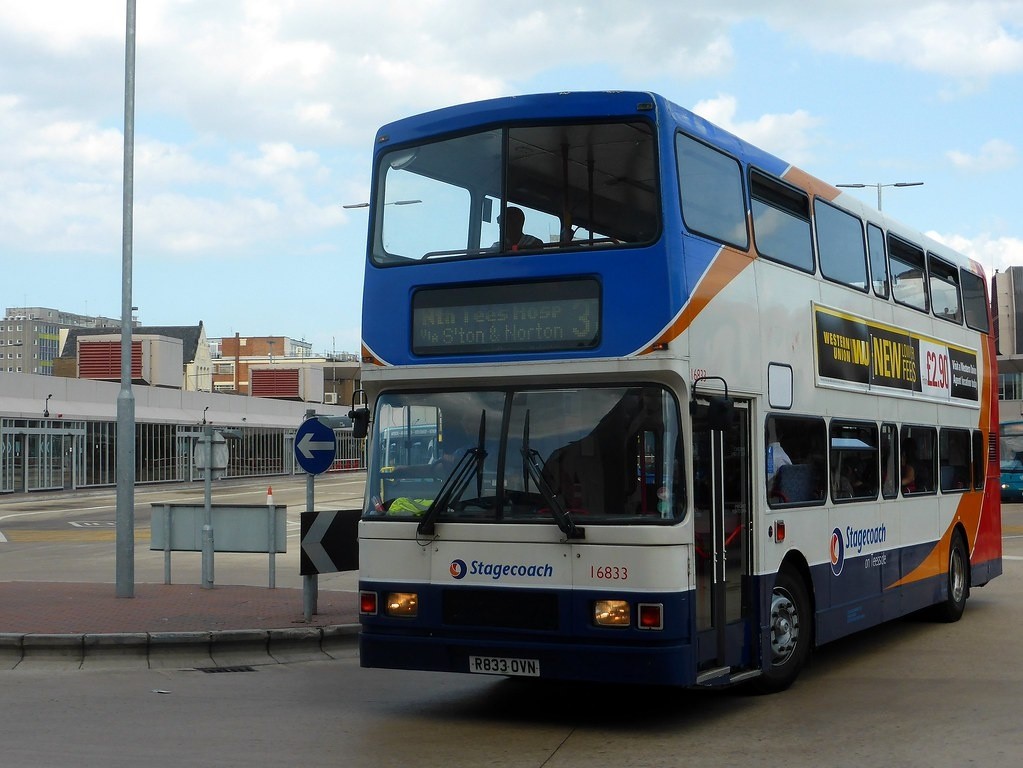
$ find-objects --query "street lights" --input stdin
[835,182,924,211]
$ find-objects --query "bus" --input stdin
[349,90,1003,697]
[996,355,1023,496]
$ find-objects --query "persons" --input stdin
[900,452,915,494]
[392,422,478,500]
[487,206,543,254]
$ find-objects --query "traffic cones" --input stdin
[267,486,274,506]
